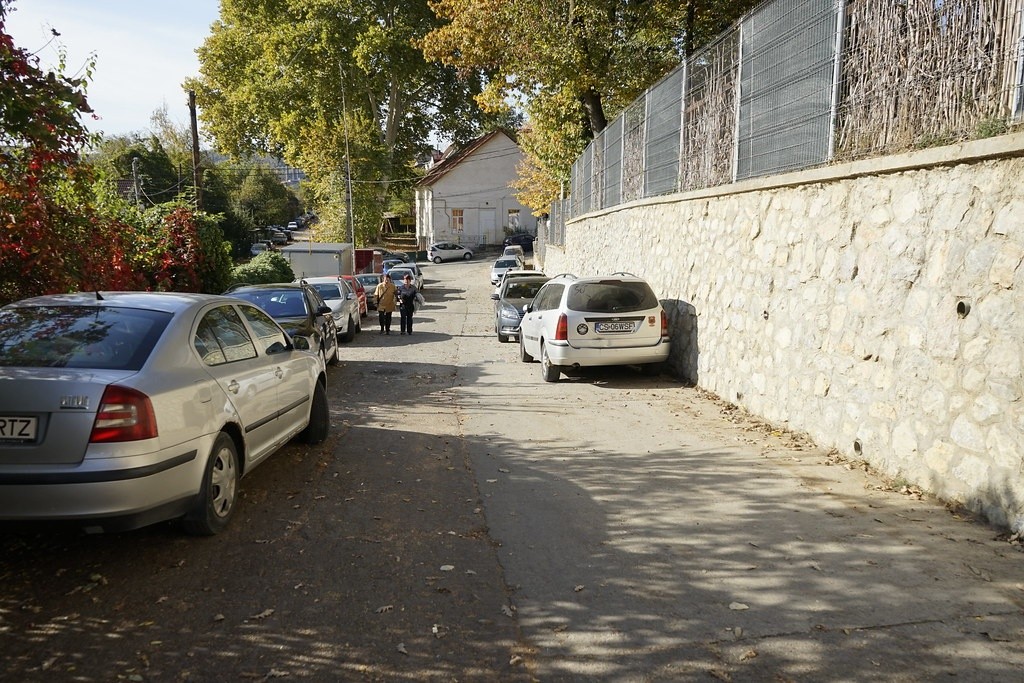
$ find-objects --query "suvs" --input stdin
[518,271,672,383]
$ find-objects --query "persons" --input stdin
[396,274,420,335]
[373,274,398,335]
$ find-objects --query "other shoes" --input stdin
[401,332,406,335]
[379,330,384,334]
[385,332,390,335]
[409,333,413,335]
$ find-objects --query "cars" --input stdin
[356,258,423,311]
[369,247,411,263]
[218,277,340,373]
[489,254,526,286]
[501,234,535,252]
[1,291,332,537]
[500,244,525,262]
[270,276,362,343]
[427,241,474,264]
[490,277,553,343]
[323,274,368,318]
[496,268,548,295]
[250,212,313,256]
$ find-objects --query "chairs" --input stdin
[281,297,306,316]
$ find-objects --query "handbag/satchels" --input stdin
[374,307,377,311]
